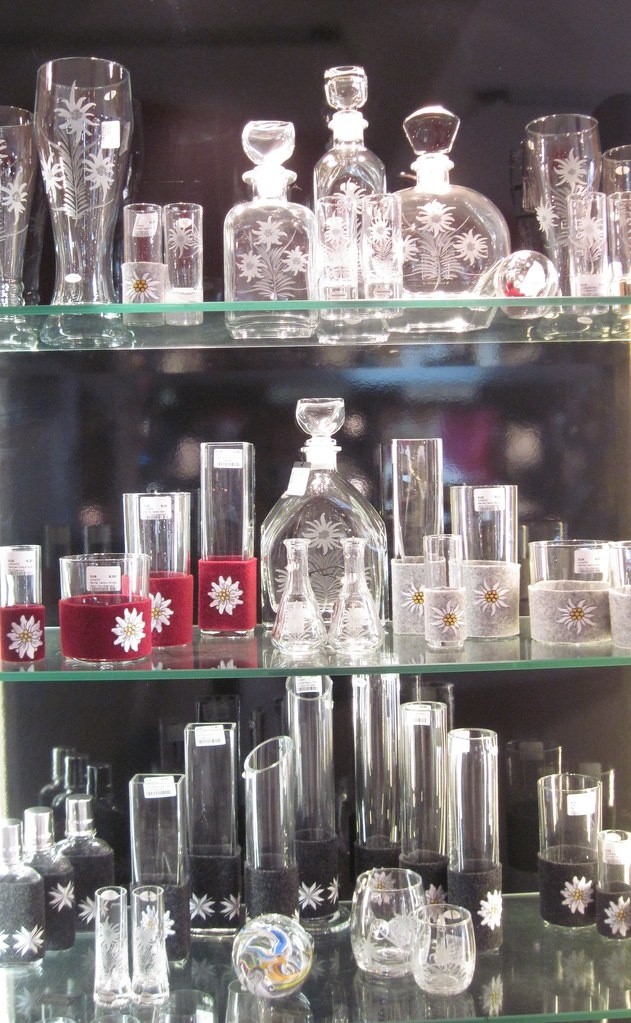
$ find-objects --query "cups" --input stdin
[126,671,340,967]
[0,105,37,348]
[93,881,172,1009]
[121,203,205,328]
[528,539,631,650]
[351,868,431,979]
[410,903,477,996]
[389,437,521,649]
[524,113,601,310]
[60,553,152,666]
[602,144,631,197]
[313,194,405,323]
[33,56,134,347]
[122,491,194,649]
[568,190,631,316]
[537,772,631,940]
[0,545,44,663]
[198,440,256,641]
[352,674,503,956]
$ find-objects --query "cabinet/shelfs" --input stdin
[0,294,631,1023]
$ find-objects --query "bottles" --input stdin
[389,106,512,333]
[38,746,122,860]
[271,537,328,653]
[0,818,46,970]
[312,64,389,344]
[327,537,385,653]
[22,805,76,951]
[222,119,319,339]
[56,793,116,933]
[260,397,387,631]
